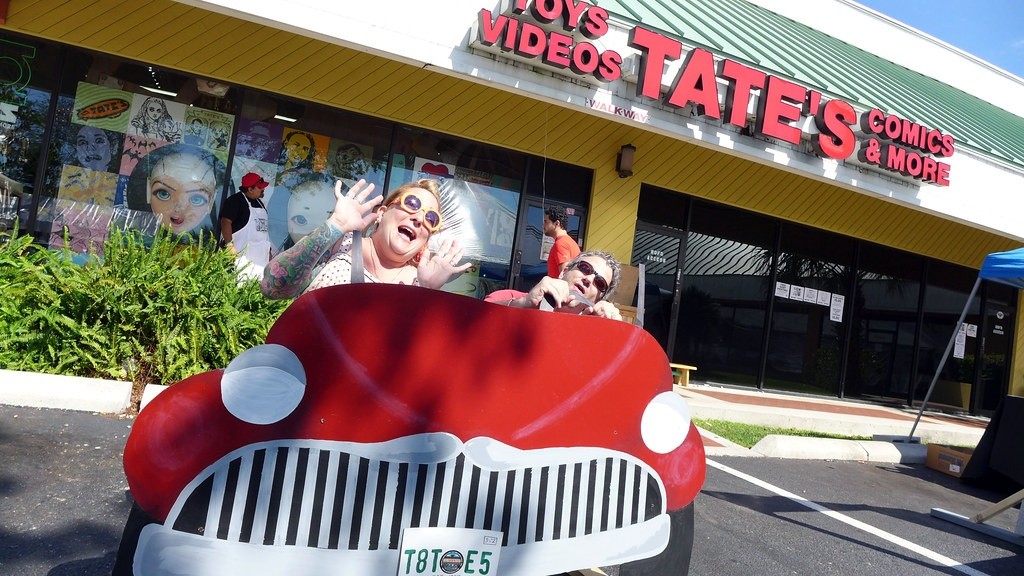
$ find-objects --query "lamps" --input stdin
[615,143,637,180]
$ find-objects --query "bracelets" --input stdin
[226,242,233,246]
[508,298,515,306]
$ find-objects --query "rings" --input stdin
[450,261,456,267]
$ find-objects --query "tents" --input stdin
[907,248,1024,442]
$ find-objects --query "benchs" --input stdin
[670,363,697,387]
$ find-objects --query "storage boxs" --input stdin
[925,443,975,478]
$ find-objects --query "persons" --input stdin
[543,207,580,278]
[484,249,623,321]
[220,173,273,289]
[261,178,472,300]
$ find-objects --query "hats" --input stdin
[242,171,270,190]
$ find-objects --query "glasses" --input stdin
[569,260,609,299]
[386,192,442,234]
[252,177,264,188]
